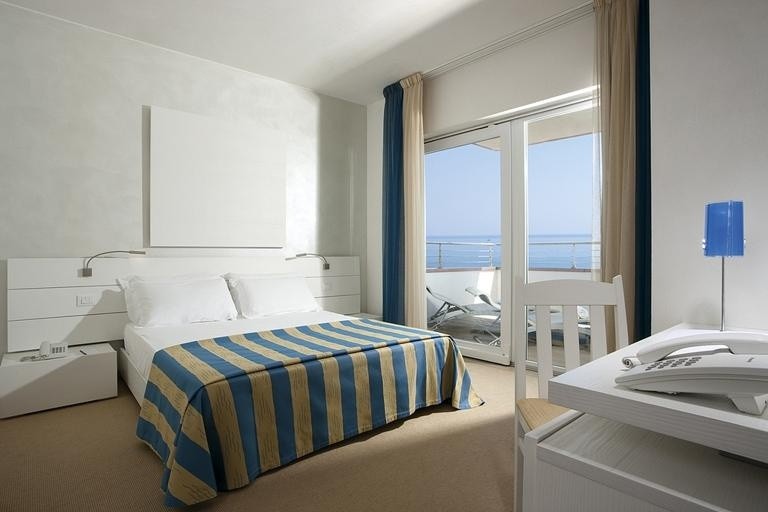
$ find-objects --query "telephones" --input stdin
[616,332,767,415]
[21,341,68,361]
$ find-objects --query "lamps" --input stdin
[704,201,746,331]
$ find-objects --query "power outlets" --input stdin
[76,295,95,306]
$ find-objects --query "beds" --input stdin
[119,273,481,493]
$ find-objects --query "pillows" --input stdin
[226,275,322,318]
[122,276,238,327]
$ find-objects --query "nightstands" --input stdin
[1,343,118,419]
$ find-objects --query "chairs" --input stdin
[513,274,629,511]
[464,287,595,348]
[425,285,501,332]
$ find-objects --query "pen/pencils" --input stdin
[80,349,87,354]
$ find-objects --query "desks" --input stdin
[524,324,767,511]
